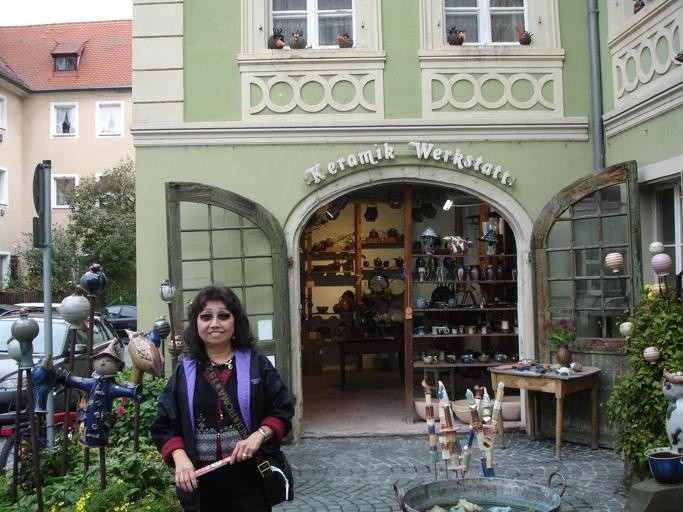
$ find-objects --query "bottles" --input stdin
[363,228,404,268]
[417,226,517,281]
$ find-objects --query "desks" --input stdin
[486,361,601,459]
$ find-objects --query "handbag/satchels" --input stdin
[256,443,303,509]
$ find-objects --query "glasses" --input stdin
[196,310,233,323]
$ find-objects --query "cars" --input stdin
[0,298,137,339]
[576,291,627,312]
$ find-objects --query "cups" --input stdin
[313,262,355,336]
[414,298,519,363]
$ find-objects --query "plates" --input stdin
[368,275,405,321]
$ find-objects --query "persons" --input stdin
[151,285,294,512]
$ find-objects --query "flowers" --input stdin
[291,28,303,40]
[543,319,576,347]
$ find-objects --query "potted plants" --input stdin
[518,30,533,44]
[446,24,466,45]
[267,27,285,48]
[338,31,352,48]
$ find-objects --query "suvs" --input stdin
[0,305,124,434]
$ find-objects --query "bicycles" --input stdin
[0,368,70,489]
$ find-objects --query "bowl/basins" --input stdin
[415,395,523,423]
[644,446,683,484]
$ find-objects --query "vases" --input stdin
[552,344,572,365]
[289,37,306,48]
[642,445,682,487]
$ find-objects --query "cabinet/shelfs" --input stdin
[300,182,525,421]
